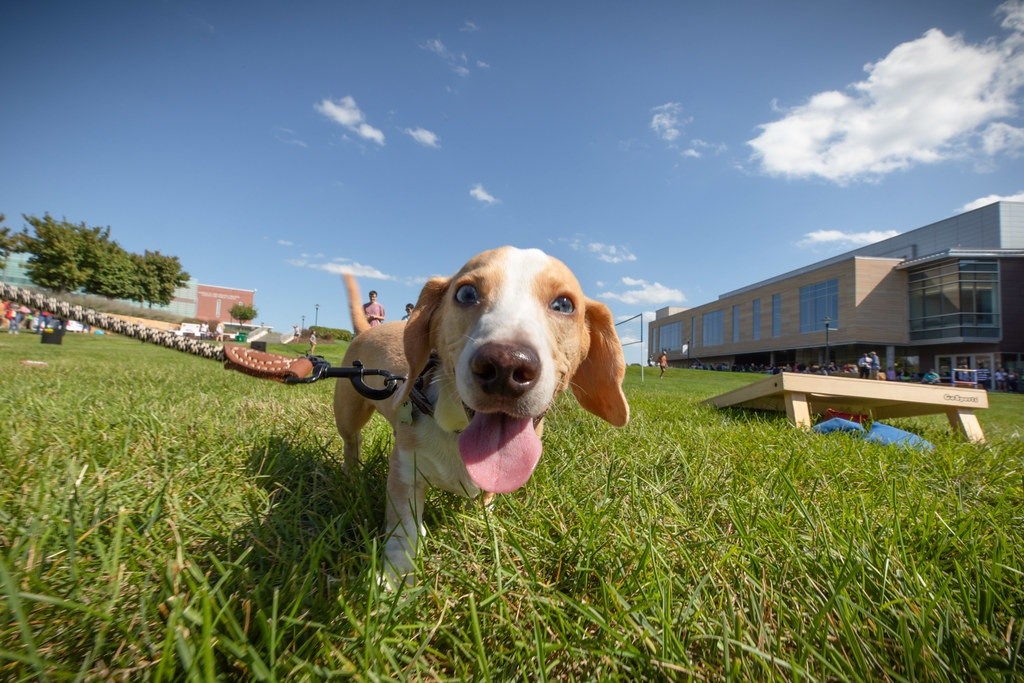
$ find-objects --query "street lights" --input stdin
[820,315,832,368]
[314,303,319,326]
[301,315,305,331]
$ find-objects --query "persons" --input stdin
[648,355,653,366]
[911,372,919,379]
[306,330,316,355]
[364,291,385,327]
[921,368,940,384]
[869,352,881,380]
[858,353,872,379]
[402,303,414,321]
[201,321,209,340]
[958,365,969,380]
[898,368,907,376]
[995,364,1019,393]
[215,321,225,341]
[0,299,68,336]
[660,351,667,379]
[710,362,858,375]
[294,326,299,343]
[977,362,988,383]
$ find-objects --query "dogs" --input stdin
[333,245,631,588]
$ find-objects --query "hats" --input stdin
[869,351,876,355]
[663,351,666,355]
